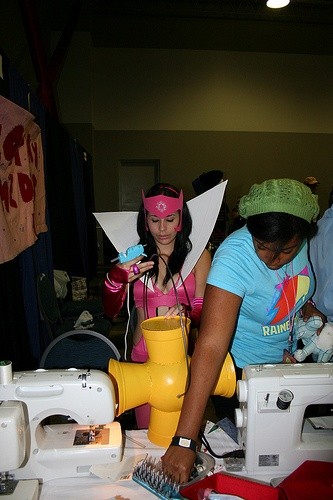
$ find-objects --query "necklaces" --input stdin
[281,264,289,282]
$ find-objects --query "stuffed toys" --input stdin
[294,316,333,363]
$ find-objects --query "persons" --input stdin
[102,183,212,430]
[163,178,327,484]
[294,204,333,322]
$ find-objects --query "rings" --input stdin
[173,304,178,309]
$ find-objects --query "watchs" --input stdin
[170,436,197,453]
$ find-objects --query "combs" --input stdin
[132,453,188,500]
